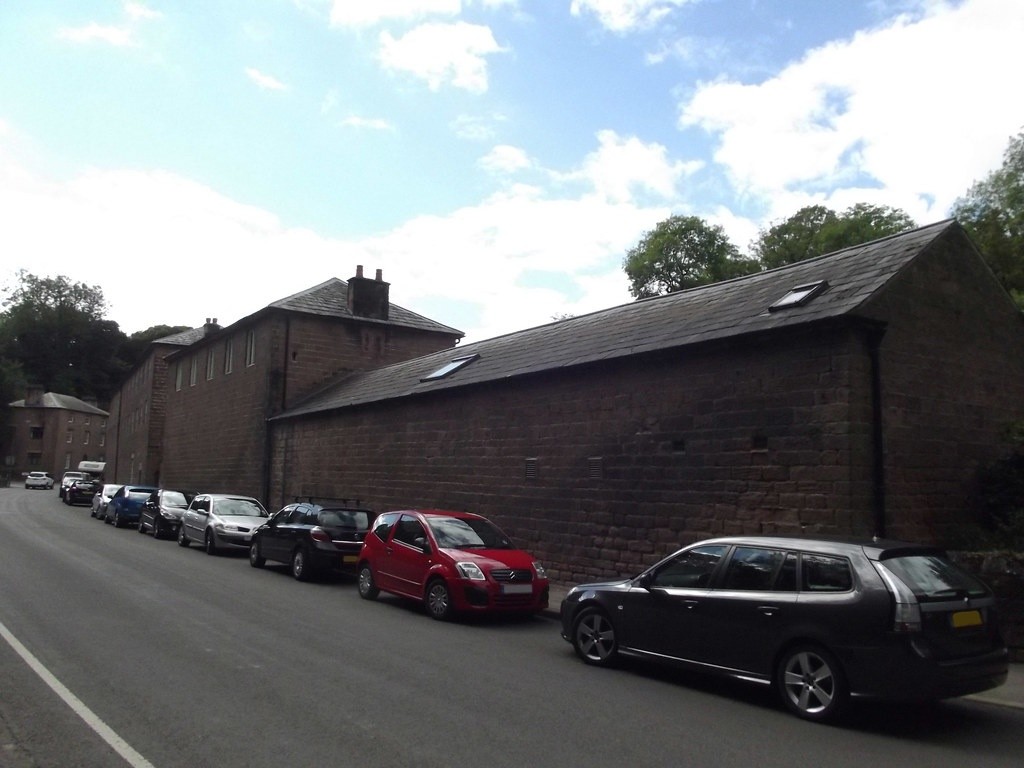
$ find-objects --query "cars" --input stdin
[356,509,550,622]
[90,484,126,519]
[138,489,200,539]
[104,485,162,528]
[248,501,379,582]
[64,479,104,506]
[176,494,275,555]
[559,532,1011,725]
[62,477,82,497]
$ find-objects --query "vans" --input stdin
[62,472,90,481]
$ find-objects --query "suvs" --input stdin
[24,471,55,490]
